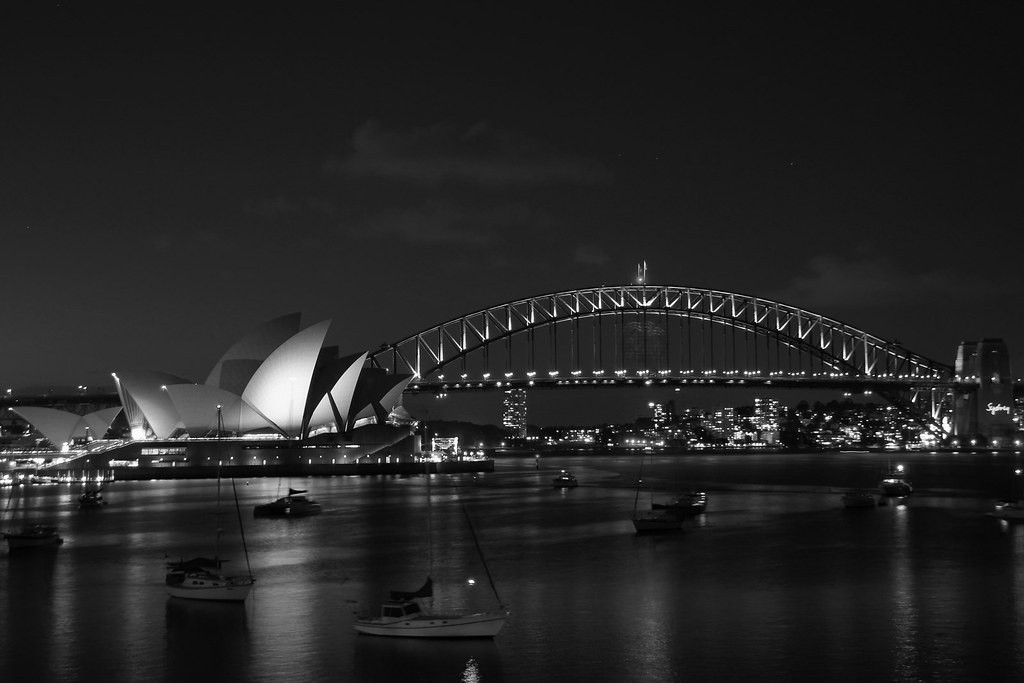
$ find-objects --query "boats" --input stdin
[79,489,104,507]
[254,489,322,519]
[841,490,875,508]
[994,500,1024,521]
[553,470,579,488]
[652,490,708,516]
[0,524,64,552]
[879,465,913,497]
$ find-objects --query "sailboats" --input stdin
[629,452,685,533]
[352,427,511,640]
[164,409,257,602]
